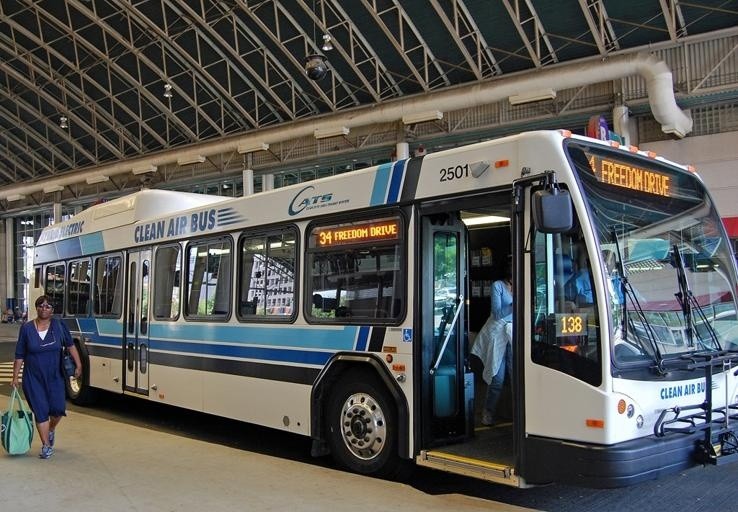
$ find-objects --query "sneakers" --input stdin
[37,445,56,460]
[480,413,495,427]
[47,429,56,449]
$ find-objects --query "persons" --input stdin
[1,305,29,324]
[552,253,580,303]
[573,250,627,305]
[9,295,82,459]
[469,254,514,426]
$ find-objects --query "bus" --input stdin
[27,128,738,500]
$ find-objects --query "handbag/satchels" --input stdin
[56,346,84,400]
[0,386,34,457]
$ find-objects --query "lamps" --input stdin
[59,113,69,129]
[321,34,334,51]
[163,83,173,98]
[304,3,327,83]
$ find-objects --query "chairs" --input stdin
[553,252,574,299]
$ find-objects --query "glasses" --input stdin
[37,304,52,309]
